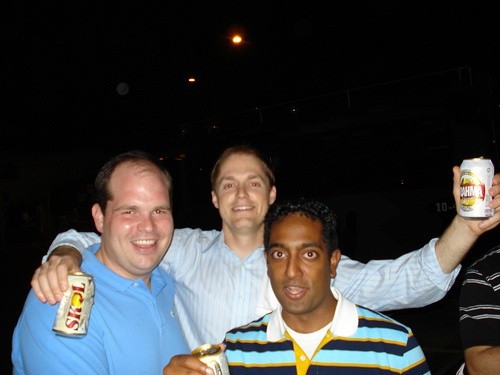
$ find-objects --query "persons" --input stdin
[457,246,500,375]
[11,152,227,375]
[32,144,500,354]
[222,200,431,375]
